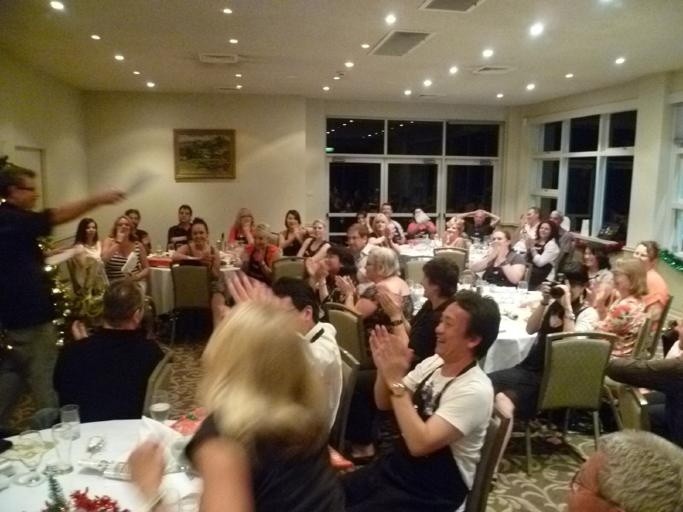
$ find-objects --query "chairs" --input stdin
[610,293,671,363]
[434,248,469,276]
[322,301,371,367]
[524,330,616,468]
[171,259,212,340]
[271,257,306,283]
[403,257,431,288]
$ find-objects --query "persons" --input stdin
[126,304,348,511]
[38,192,683,468]
[340,290,500,511]
[565,429,683,512]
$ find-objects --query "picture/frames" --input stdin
[172,127,236,181]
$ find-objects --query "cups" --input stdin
[408,232,490,252]
[52,422,71,473]
[62,403,79,440]
[515,281,528,303]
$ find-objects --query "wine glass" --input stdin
[410,283,424,311]
[14,428,45,486]
[139,241,247,269]
[148,388,170,431]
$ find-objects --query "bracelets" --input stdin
[144,490,169,509]
[390,320,404,326]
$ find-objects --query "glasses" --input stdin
[569,471,621,507]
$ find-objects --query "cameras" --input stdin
[548,273,566,298]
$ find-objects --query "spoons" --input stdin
[80,436,106,464]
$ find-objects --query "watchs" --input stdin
[388,383,406,398]
